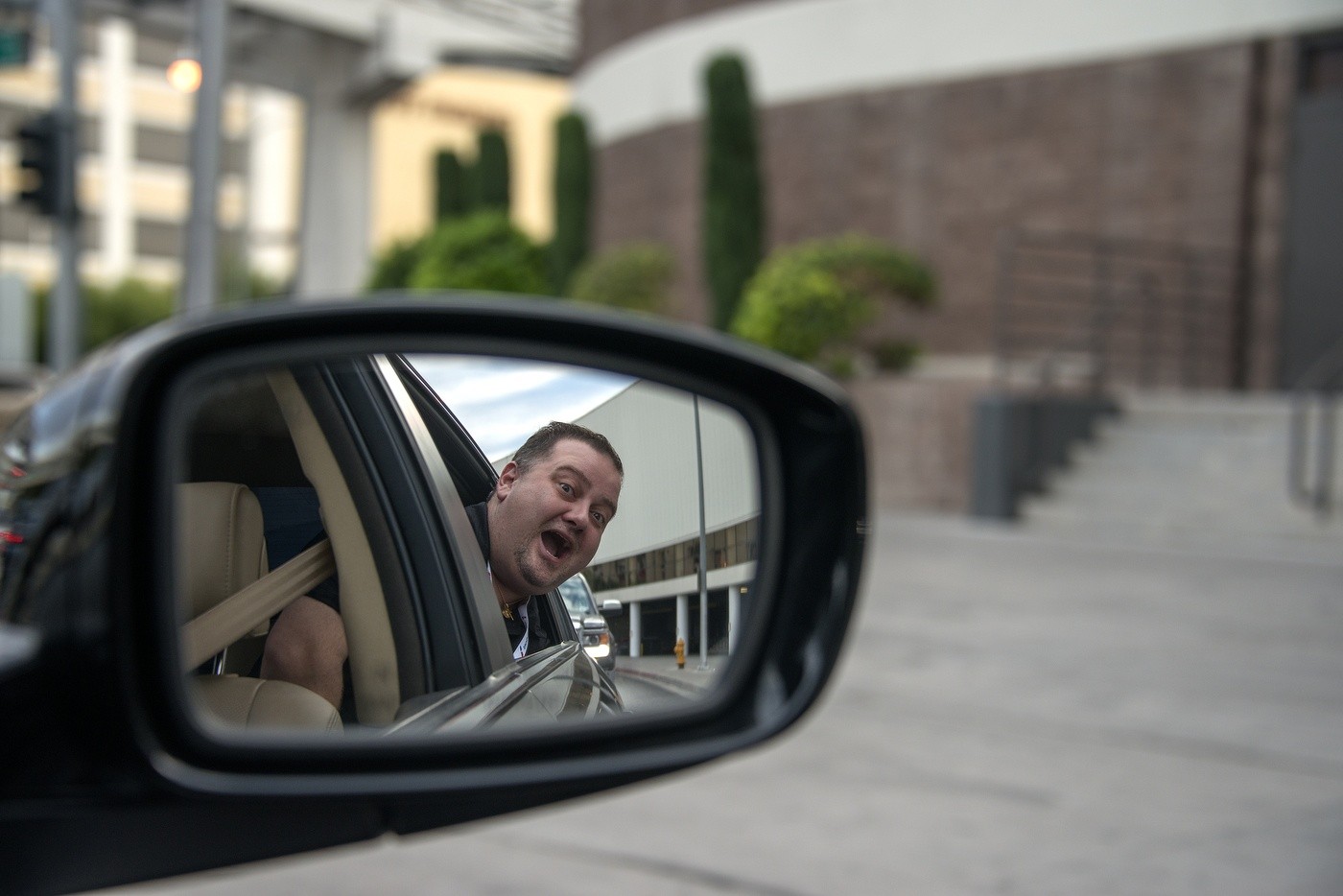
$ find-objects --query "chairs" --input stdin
[173,477,341,746]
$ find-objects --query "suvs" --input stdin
[549,569,629,680]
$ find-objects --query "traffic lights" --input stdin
[18,115,65,216]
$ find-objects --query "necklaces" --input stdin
[492,576,527,620]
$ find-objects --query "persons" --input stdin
[262,415,628,726]
